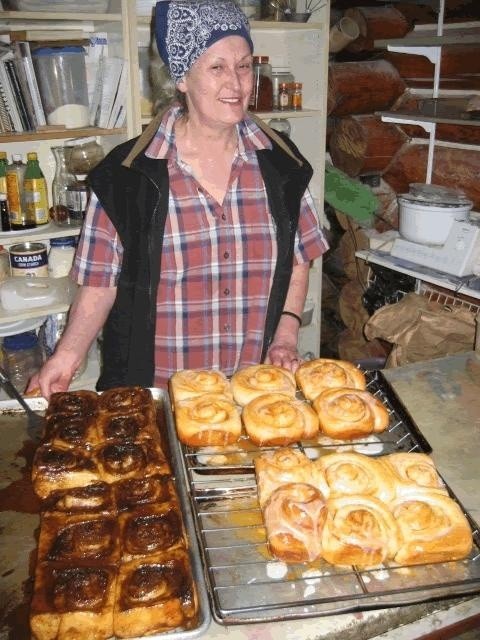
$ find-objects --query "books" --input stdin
[0,25,127,135]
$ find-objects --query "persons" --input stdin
[25,0,331,401]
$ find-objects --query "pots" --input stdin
[392,182,471,244]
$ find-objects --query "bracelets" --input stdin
[279,311,302,327]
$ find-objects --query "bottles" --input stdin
[247,55,303,111]
[32,45,93,132]
[50,136,105,228]
[48,237,78,278]
[2,333,44,393]
[0,152,51,229]
[0,243,12,285]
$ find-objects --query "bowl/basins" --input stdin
[288,12,313,24]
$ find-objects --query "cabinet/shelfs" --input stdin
[352,0,479,301]
[129,0,332,366]
[1,0,128,398]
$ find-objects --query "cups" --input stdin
[330,16,359,55]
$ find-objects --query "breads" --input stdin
[170,357,389,437]
[30,388,197,636]
[254,446,474,566]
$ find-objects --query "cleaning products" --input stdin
[6,154,28,229]
[23,151,49,225]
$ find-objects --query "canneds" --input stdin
[9,242,49,289]
[64,135,103,174]
[280,82,302,110]
